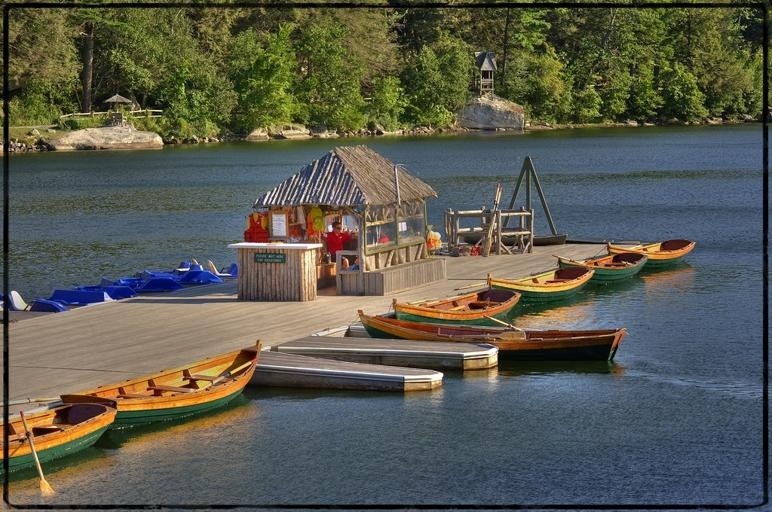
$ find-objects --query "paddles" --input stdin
[19,411,53,494]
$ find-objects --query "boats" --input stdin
[1,338,262,472]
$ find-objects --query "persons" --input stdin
[325,221,352,267]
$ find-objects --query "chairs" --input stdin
[315,239,338,290]
